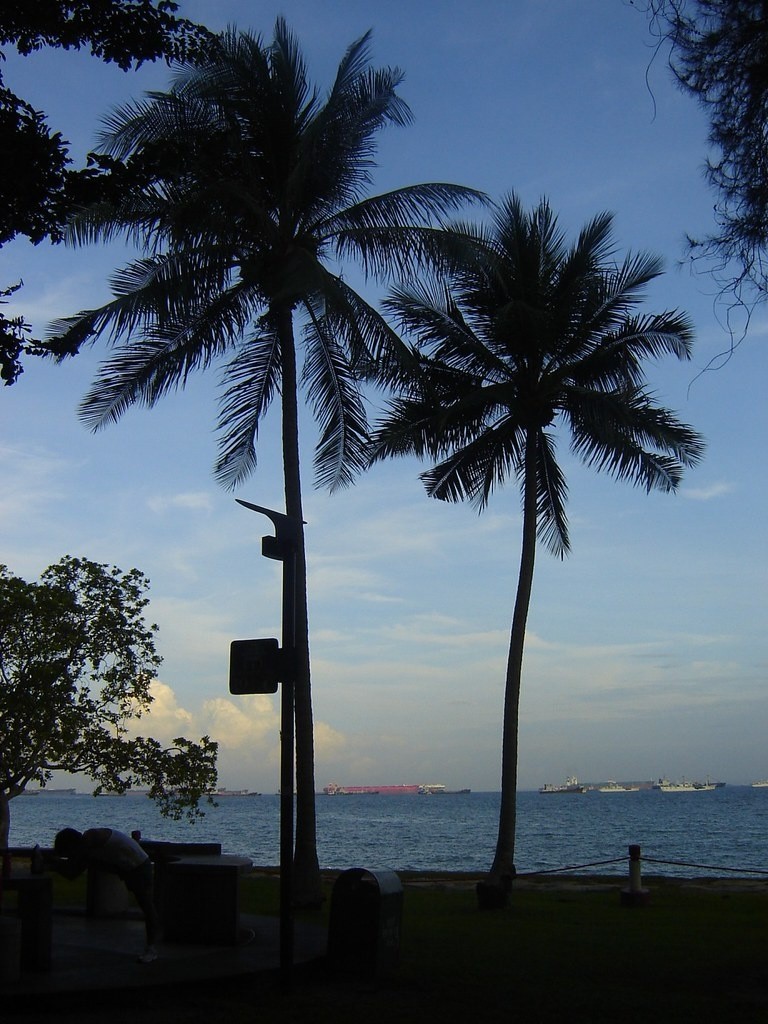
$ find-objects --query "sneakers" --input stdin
[139,944,158,963]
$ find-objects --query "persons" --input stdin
[54,827,161,963]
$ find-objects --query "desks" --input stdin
[0,866,55,973]
[152,852,253,943]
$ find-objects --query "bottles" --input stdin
[2,851,12,877]
